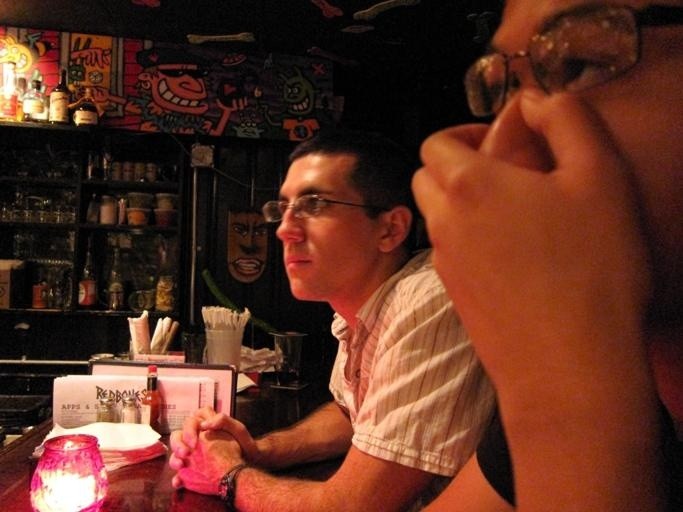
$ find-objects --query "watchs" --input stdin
[217,463,256,512]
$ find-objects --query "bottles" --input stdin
[29,431,111,512]
[0,57,180,320]
[97,364,165,437]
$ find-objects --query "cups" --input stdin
[201,328,244,370]
[268,328,309,386]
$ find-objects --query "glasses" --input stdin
[261,196,375,225]
[464,0,680,121]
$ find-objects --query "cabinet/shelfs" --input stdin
[0,121,192,361]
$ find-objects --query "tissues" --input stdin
[34,421,169,512]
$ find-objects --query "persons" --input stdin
[423,0,683,512]
[227,206,269,284]
[168,124,499,512]
